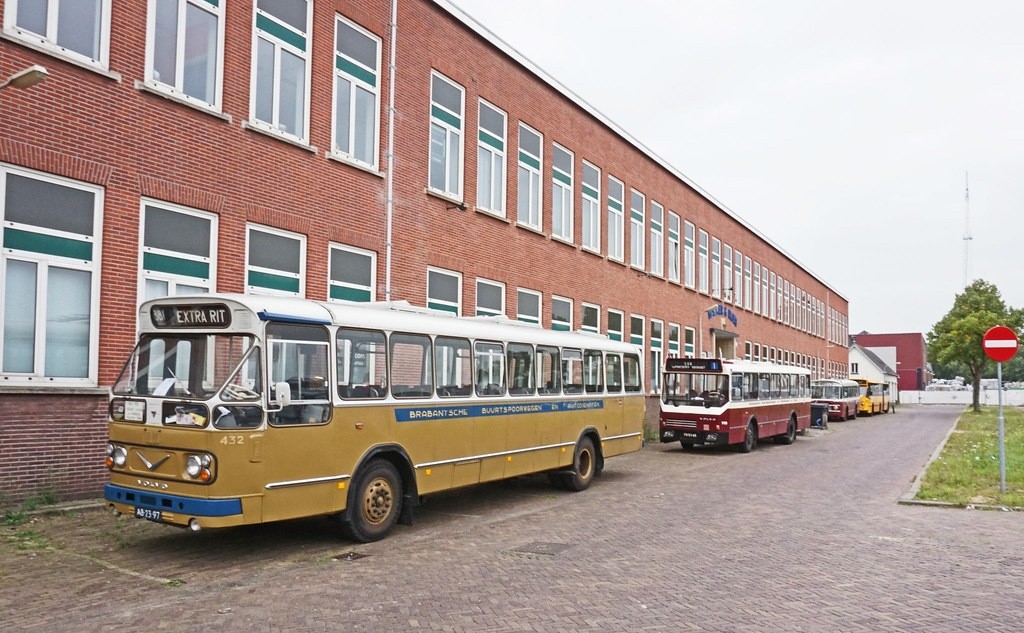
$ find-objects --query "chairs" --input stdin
[270,384,585,424]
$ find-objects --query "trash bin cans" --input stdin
[810,403,829,427]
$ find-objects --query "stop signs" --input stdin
[983,326,1019,362]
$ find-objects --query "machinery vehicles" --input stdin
[656,357,812,453]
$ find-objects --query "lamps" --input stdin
[1,63,50,91]
[446,203,467,212]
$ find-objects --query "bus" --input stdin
[809,379,860,422]
[850,378,891,416]
[100,290,645,544]
